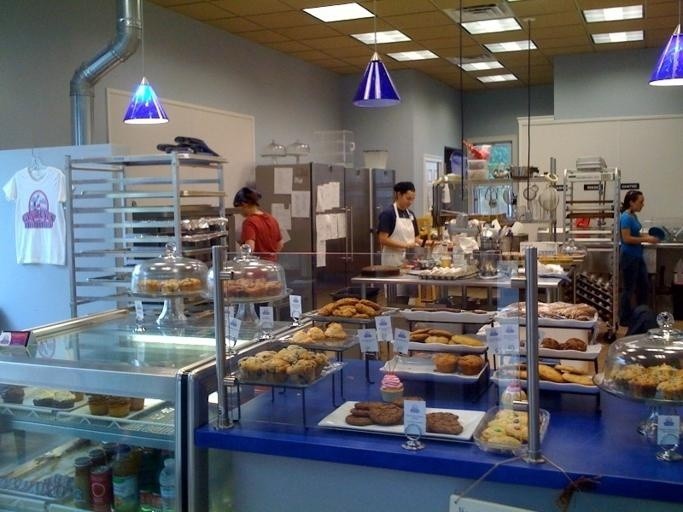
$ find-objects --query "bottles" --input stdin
[74,441,178,512]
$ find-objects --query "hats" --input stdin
[393,181,414,192]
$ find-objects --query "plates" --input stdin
[520,344,602,360]
[495,311,598,329]
[472,404,550,455]
[318,399,486,442]
[490,365,600,394]
[398,309,495,324]
[3,396,88,412]
[391,340,488,354]
[379,355,489,384]
[71,398,168,418]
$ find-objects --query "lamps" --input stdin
[650,2,683,87]
[123,2,171,130]
[350,3,404,107]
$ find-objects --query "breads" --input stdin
[456,355,483,374]
[612,365,683,400]
[238,345,328,385]
[141,278,201,293]
[432,352,456,371]
[224,278,278,296]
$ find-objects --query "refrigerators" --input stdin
[256,164,370,312]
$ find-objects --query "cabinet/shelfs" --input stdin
[561,155,625,326]
[350,237,572,344]
[64,150,233,326]
[430,158,554,273]
[194,335,683,512]
[0,290,298,512]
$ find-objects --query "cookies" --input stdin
[480,410,528,447]
[504,364,593,385]
[410,328,483,346]
[428,413,462,434]
[318,297,382,319]
[347,396,424,435]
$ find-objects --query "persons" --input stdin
[376,182,417,304]
[234,188,282,322]
[617,190,660,327]
